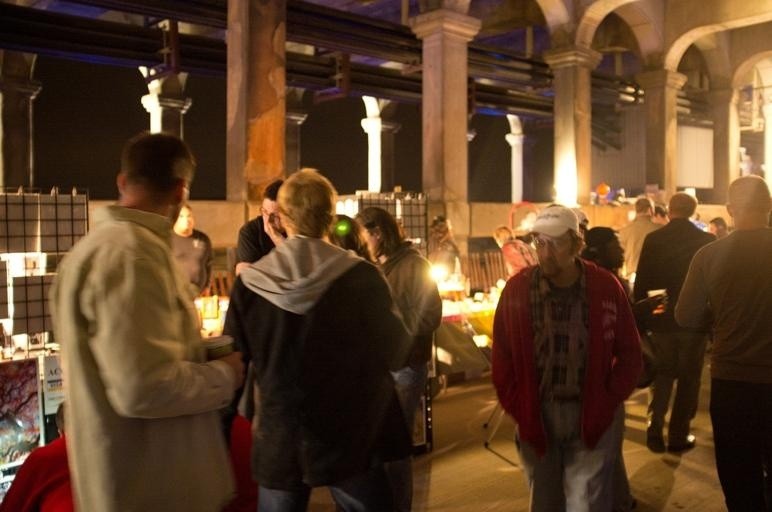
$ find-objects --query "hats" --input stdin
[524,204,579,240]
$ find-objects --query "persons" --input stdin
[570,209,589,242]
[169,204,214,298]
[653,206,667,227]
[488,203,643,512]
[356,207,443,431]
[710,218,727,241]
[617,198,665,283]
[492,227,539,277]
[41,136,243,511]
[585,228,670,319]
[330,215,368,269]
[215,167,413,511]
[235,180,288,277]
[635,193,717,453]
[674,175,772,511]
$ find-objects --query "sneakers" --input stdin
[645,421,666,454]
[668,433,699,453]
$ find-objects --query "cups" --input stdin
[207,335,234,360]
[647,288,667,314]
